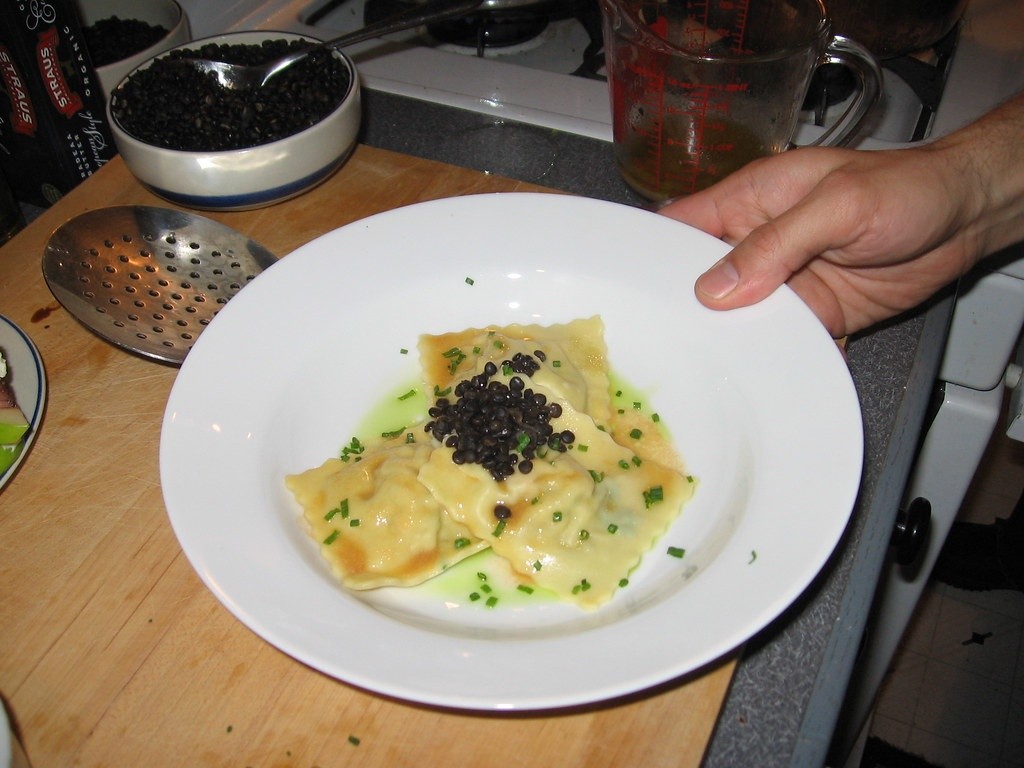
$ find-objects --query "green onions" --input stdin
[323,277,693,607]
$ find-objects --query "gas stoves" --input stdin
[234,0,1024,390]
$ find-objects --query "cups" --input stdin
[602,0,883,205]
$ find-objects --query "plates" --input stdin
[158,193,865,712]
[0,314,48,491]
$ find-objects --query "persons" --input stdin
[660,91,1024,338]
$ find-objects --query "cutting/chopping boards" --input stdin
[0,143,745,767]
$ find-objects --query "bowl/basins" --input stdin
[75,0,192,101]
[107,36,360,205]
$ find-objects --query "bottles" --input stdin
[0,0,118,207]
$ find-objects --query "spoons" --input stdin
[176,0,482,87]
